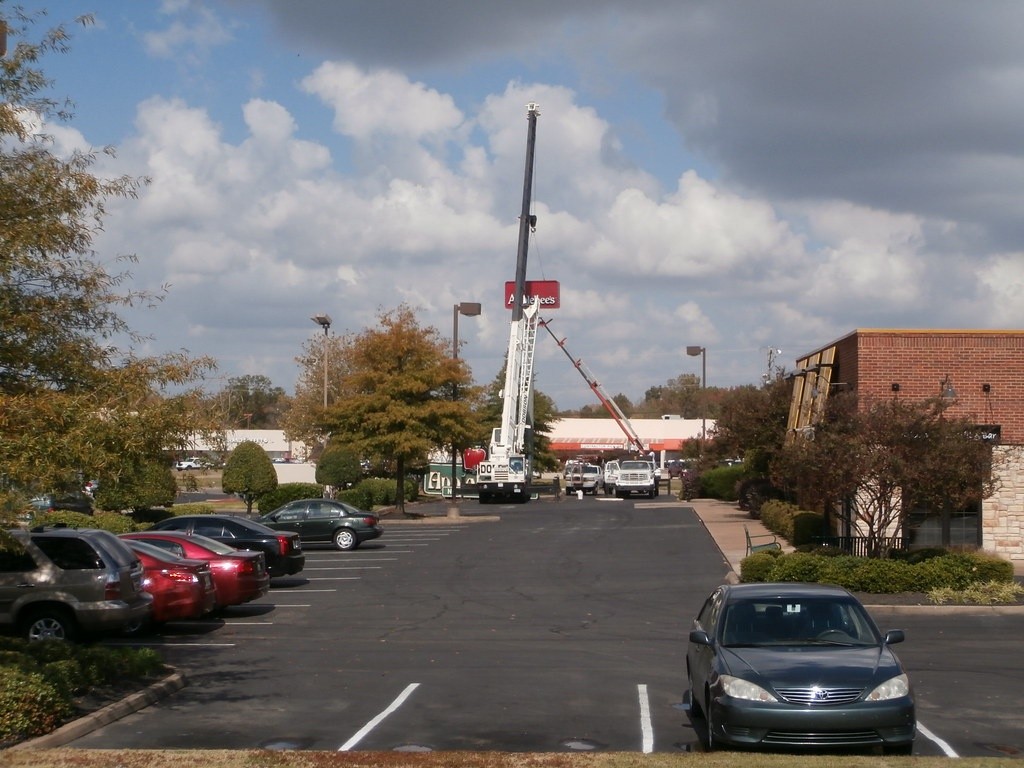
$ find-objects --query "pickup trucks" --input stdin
[566,465,613,496]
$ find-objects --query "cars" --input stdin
[117,532,270,618]
[19,478,100,522]
[118,537,216,640]
[204,461,226,470]
[253,499,384,551]
[685,579,917,756]
[142,517,304,581]
[273,456,306,463]
[668,462,692,479]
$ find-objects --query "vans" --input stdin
[563,459,585,478]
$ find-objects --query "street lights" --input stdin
[452,301,482,509]
[310,313,331,449]
[686,345,706,450]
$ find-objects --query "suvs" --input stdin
[176,457,206,472]
[0,523,155,651]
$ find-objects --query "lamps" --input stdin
[811,375,848,397]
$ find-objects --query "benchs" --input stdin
[743,523,781,557]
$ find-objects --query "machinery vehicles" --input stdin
[538,316,671,499]
[475,101,543,503]
